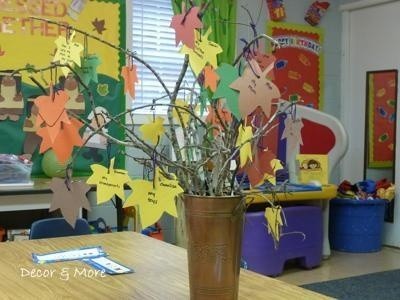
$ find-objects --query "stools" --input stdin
[243,204,326,279]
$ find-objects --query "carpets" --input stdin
[298,265,399,296]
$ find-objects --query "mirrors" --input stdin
[362,69,399,224]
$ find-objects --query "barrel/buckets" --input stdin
[328,198,386,255]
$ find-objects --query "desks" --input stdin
[245,183,339,260]
[1,231,331,296]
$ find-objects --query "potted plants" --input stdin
[9,1,312,296]
